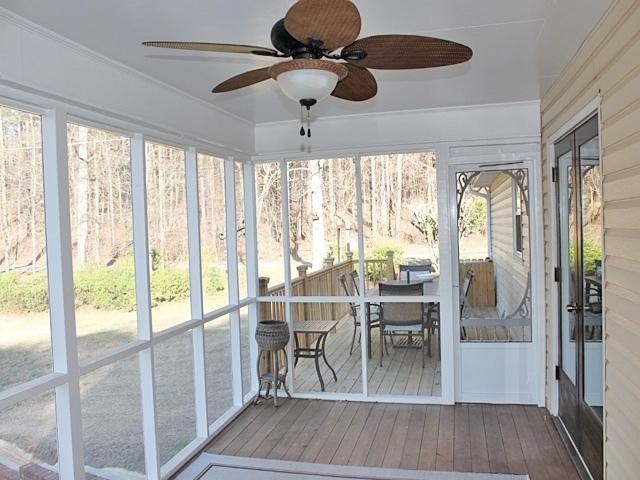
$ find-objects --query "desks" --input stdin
[584,275,602,304]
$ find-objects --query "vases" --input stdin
[253,321,292,407]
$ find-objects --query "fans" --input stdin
[142,0,473,100]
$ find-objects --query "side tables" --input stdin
[292,320,338,391]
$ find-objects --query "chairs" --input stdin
[596,259,602,304]
[571,272,602,340]
[338,264,473,368]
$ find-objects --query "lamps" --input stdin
[268,53,348,137]
